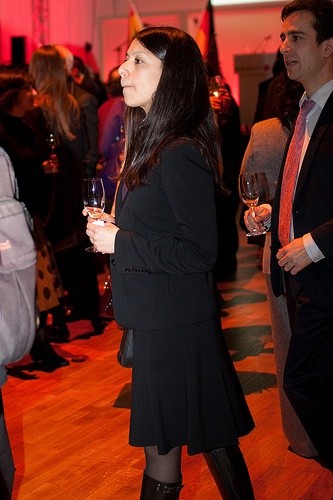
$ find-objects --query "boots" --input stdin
[140,471,184,500]
[203,442,255,500]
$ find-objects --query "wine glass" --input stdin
[239,172,266,237]
[82,178,106,253]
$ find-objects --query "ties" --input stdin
[278,98,316,246]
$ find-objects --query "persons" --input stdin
[82,27,256,499]
[244,0,333,472]
[236,42,315,459]
[0,148,19,500]
[0,38,278,371]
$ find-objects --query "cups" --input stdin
[211,76,223,98]
[46,134,59,154]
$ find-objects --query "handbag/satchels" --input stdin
[117,328,134,368]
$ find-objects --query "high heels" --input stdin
[30,338,68,366]
